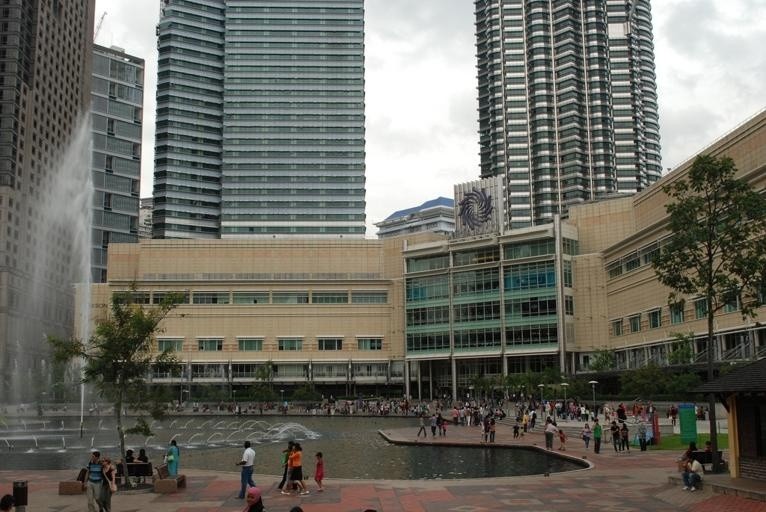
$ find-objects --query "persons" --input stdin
[411,398,656,456]
[4,402,98,413]
[234,441,257,499]
[84,438,179,512]
[0,494,14,511]
[277,441,325,495]
[167,398,412,416]
[681,441,728,491]
[244,488,266,512]
[666,404,709,427]
[291,506,302,512]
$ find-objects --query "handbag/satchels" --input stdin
[109,482,118,493]
[167,456,174,461]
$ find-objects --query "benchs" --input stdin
[153,463,188,494]
[677,451,728,473]
[58,465,88,496]
[115,461,152,488]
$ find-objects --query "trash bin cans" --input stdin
[193,402,199,407]
[13,480,28,505]
[284,405,288,410]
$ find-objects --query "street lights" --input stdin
[279,389,284,406]
[232,389,237,409]
[560,382,571,419]
[588,381,599,420]
[537,383,545,406]
